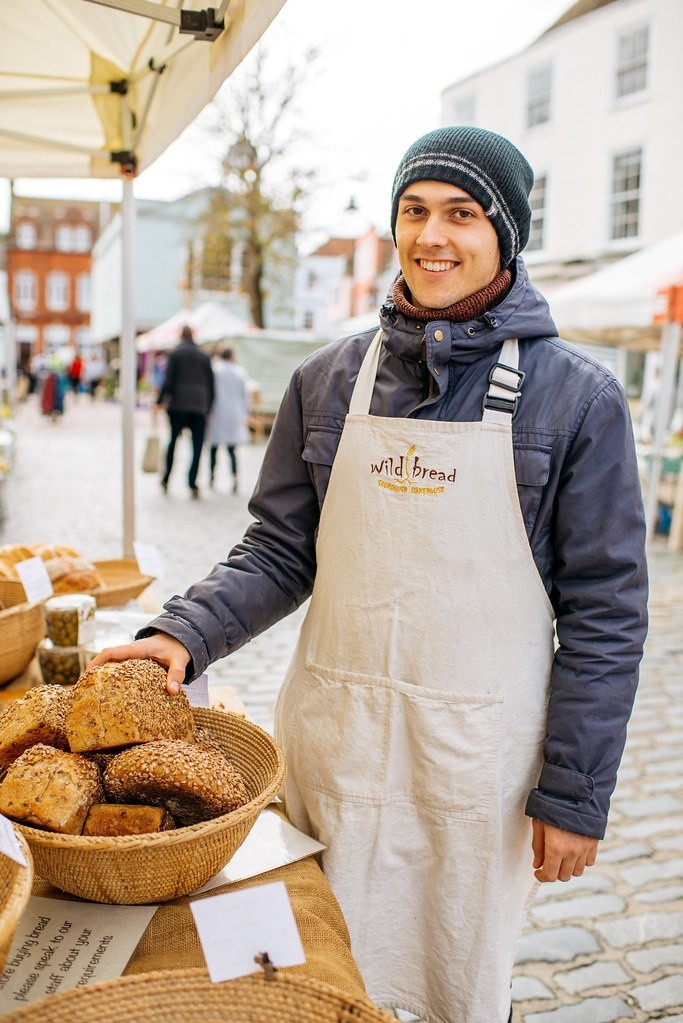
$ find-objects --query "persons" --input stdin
[89,126,647,1023]
[155,325,215,493]
[87,355,102,396]
[41,371,65,422]
[68,356,82,391]
[204,348,249,493]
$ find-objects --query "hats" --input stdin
[389,126,533,272]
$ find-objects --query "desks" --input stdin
[0,789,367,1023]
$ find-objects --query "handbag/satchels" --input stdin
[143,407,160,473]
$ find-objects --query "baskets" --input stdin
[51,559,153,610]
[0,966,401,1023]
[0,826,34,981]
[0,704,289,904]
[0,579,47,684]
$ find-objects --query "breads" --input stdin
[0,658,248,836]
[0,543,102,595]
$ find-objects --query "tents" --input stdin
[135,302,252,352]
[0,0,289,563]
[547,228,683,539]
